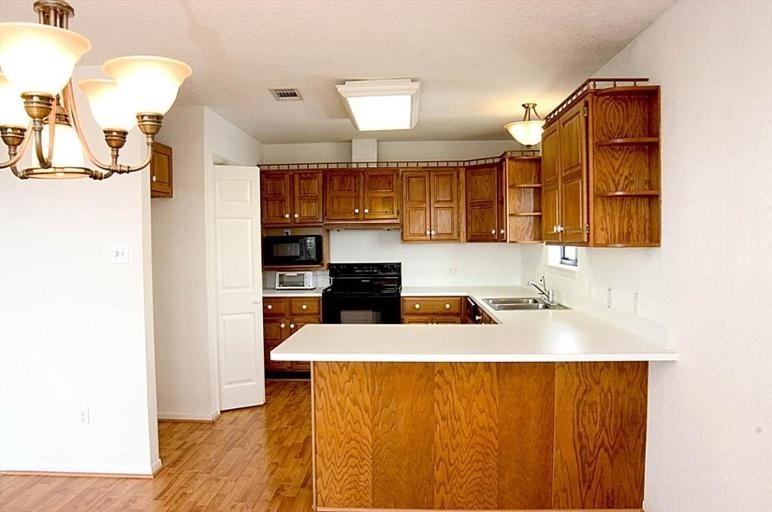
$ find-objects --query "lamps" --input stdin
[0,1,195,181]
[503,103,549,146]
[333,77,423,135]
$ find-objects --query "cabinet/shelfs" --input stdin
[261,296,318,376]
[509,156,542,244]
[542,95,594,244]
[399,166,463,242]
[592,87,661,248]
[148,139,175,199]
[323,168,399,224]
[261,171,323,224]
[465,299,488,322]
[405,297,465,325]
[465,166,507,241]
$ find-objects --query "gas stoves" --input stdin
[323,280,401,297]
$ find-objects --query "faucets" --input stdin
[540,275,548,290]
[527,281,554,303]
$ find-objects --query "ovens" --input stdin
[322,297,401,324]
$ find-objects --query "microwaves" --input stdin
[275,270,318,290]
[262,234,323,265]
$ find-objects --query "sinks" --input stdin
[481,297,558,304]
[487,304,571,311]
